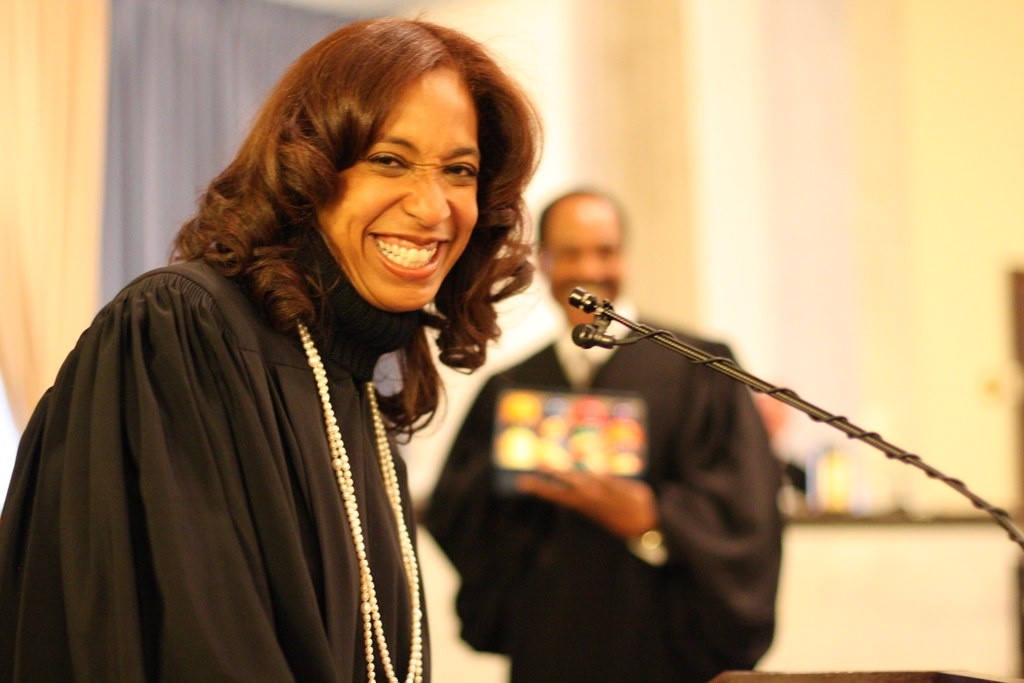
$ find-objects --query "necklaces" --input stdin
[296,322,435,683]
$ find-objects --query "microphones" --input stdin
[571,323,616,348]
[569,286,1024,548]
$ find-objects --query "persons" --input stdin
[420,192,787,683]
[0,18,546,683]
